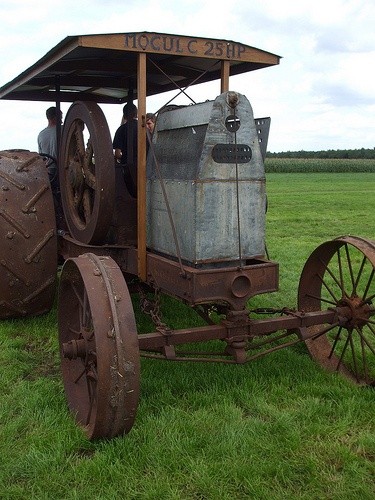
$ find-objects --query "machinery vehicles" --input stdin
[0,31,375,443]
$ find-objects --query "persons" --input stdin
[37,107,64,188]
[112,103,152,199]
[146,113,157,135]
